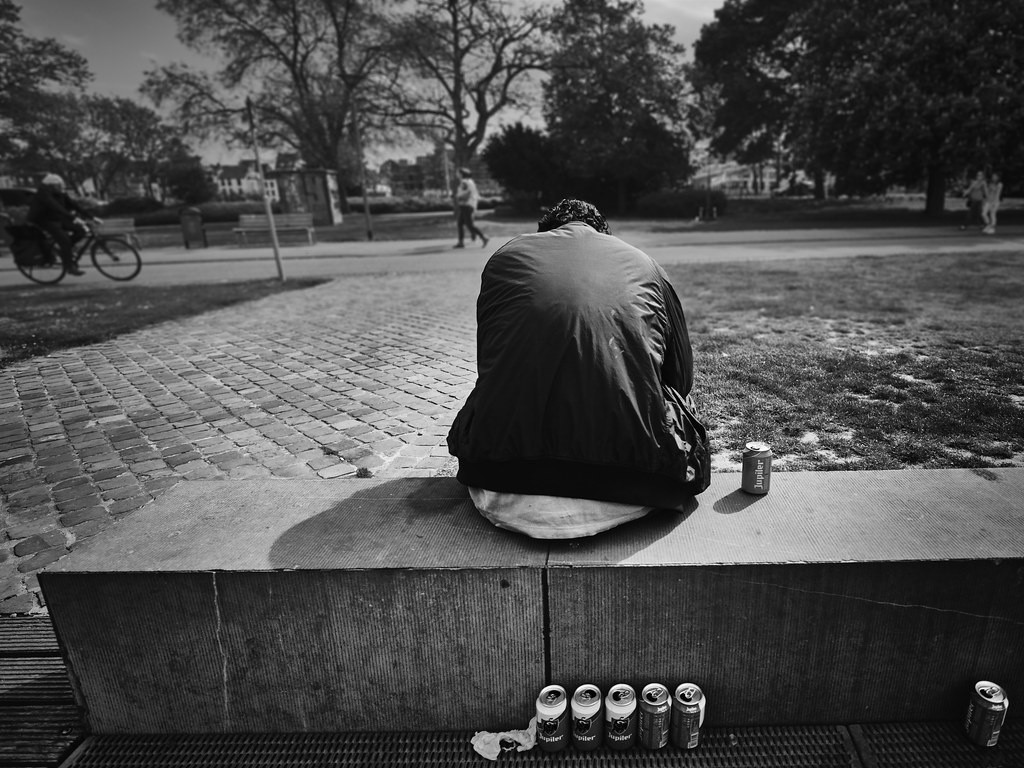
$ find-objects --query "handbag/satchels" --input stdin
[10,223,56,267]
[966,193,972,207]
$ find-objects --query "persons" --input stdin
[451,167,489,248]
[959,172,1002,234]
[446,200,711,540]
[26,174,105,275]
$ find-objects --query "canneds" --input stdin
[536,685,570,752]
[742,442,772,494]
[964,680,1009,747]
[671,682,706,749]
[605,684,638,751]
[571,683,603,750]
[638,682,672,748]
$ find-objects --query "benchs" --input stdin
[36,467,1023,736]
[232,214,316,246]
[69,218,142,251]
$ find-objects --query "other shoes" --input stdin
[983,226,995,234]
[483,237,488,248]
[958,226,967,231]
[453,243,464,248]
[69,268,86,275]
[979,224,986,229]
[71,244,78,252]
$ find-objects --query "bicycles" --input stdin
[13,216,142,285]
